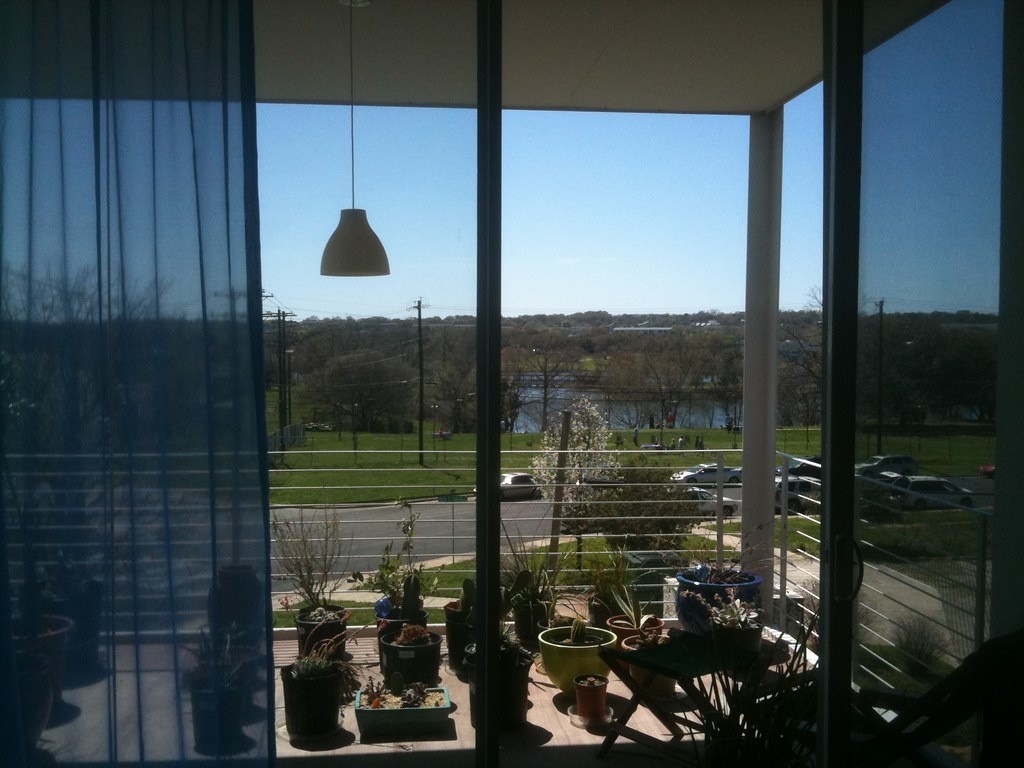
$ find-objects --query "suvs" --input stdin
[854,454,918,478]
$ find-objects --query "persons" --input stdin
[678,435,687,457]
[439,427,446,440]
[694,434,704,456]
[725,413,734,432]
[632,428,639,446]
[650,434,676,450]
[666,412,674,428]
[648,414,654,429]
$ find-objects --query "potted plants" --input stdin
[1,398,78,755]
[443,568,532,672]
[674,512,807,635]
[378,624,444,691]
[274,596,388,747]
[463,620,535,735]
[496,500,587,642]
[270,484,355,664]
[204,561,267,668]
[568,673,616,729]
[354,669,453,741]
[605,582,666,671]
[345,492,429,637]
[537,617,619,698]
[621,629,681,700]
[176,621,262,755]
[573,537,662,628]
[13,456,142,692]
[677,586,765,649]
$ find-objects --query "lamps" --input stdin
[319,1,395,277]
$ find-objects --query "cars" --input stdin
[676,487,737,519]
[775,454,822,478]
[774,475,822,495]
[669,462,743,489]
[473,472,544,501]
[887,476,977,509]
